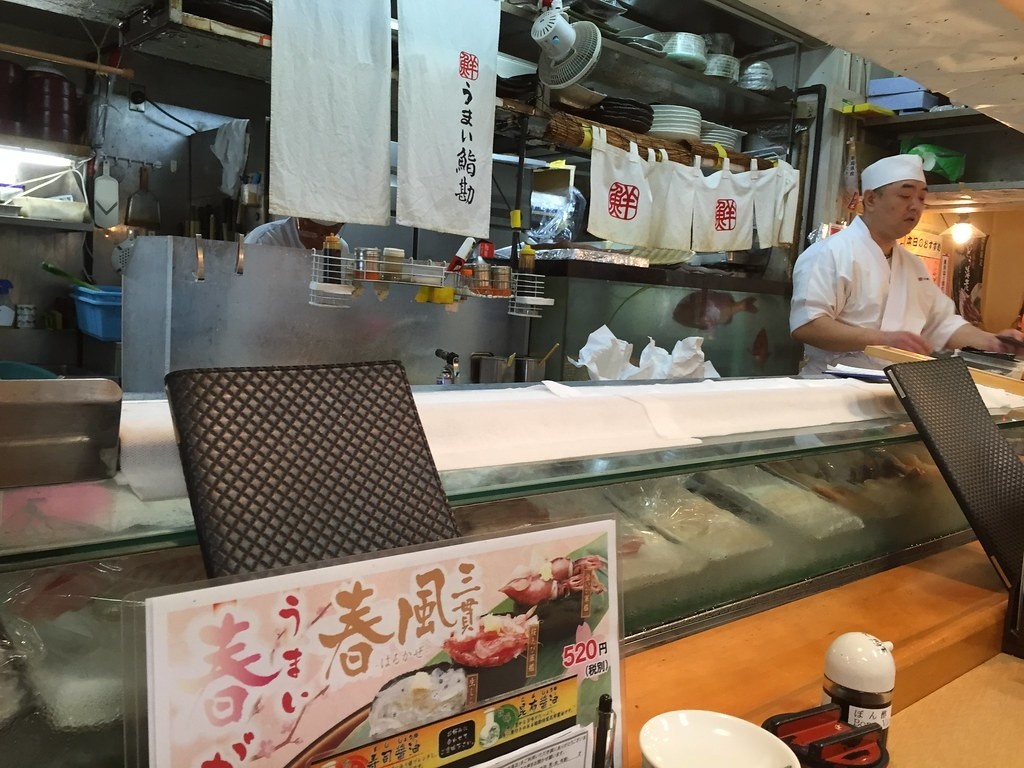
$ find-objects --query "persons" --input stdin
[242,215,351,253]
[789,154,1024,448]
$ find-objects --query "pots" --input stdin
[471,351,546,382]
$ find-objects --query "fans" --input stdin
[531,0,602,89]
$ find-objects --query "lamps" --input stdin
[939,214,987,245]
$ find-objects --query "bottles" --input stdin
[478,707,501,748]
[472,264,489,288]
[824,631,896,749]
[354,247,368,279]
[460,269,471,290]
[322,232,342,284]
[366,250,380,280]
[490,265,510,288]
[518,245,535,273]
[382,247,404,281]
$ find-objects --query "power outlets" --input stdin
[129,84,145,112]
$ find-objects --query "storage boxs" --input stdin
[473,239,494,257]
[866,77,939,111]
[533,165,576,201]
[69,285,123,341]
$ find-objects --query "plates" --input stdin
[582,96,749,151]
[616,31,740,83]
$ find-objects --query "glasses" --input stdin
[296,217,344,238]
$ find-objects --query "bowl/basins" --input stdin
[638,709,800,768]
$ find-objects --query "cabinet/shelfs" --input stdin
[857,108,1024,206]
[470,0,805,385]
[0,0,123,363]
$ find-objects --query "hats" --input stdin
[860,154,925,193]
[310,217,337,226]
